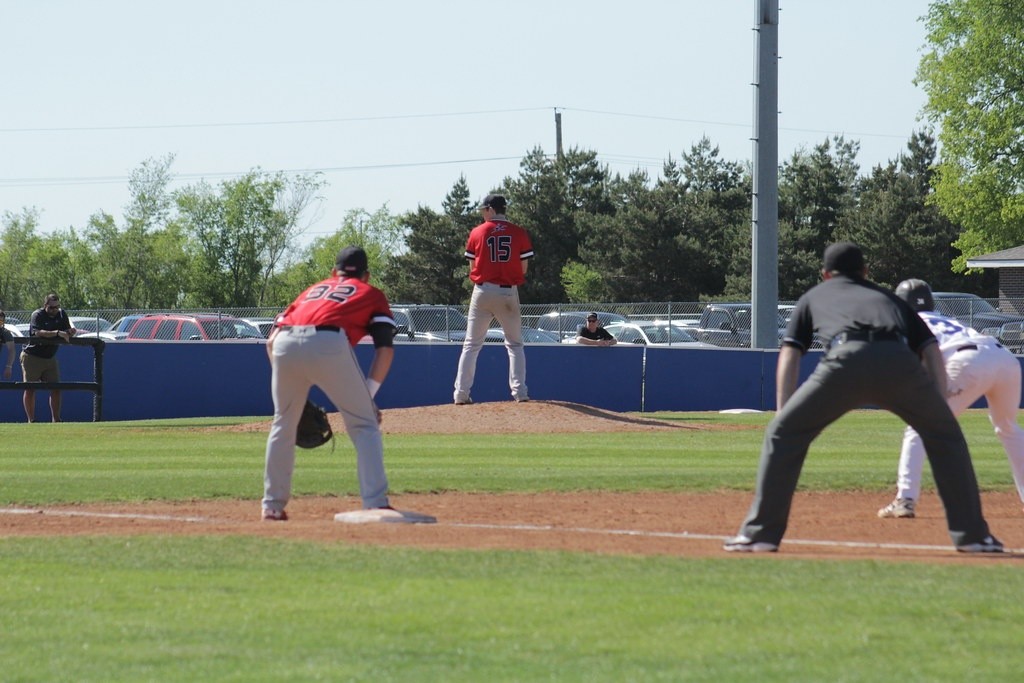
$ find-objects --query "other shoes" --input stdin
[455,397,473,405]
[517,397,529,403]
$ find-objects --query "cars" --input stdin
[0,290,1024,353]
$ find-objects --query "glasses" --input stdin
[588,320,597,323]
[46,304,60,310]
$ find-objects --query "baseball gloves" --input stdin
[294,397,333,449]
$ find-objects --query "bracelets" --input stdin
[57,329,59,336]
[6,365,12,368]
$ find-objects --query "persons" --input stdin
[262,244,399,520]
[576,312,617,346]
[20,293,76,422]
[877,275,1024,517]
[453,193,534,405]
[0,310,16,378]
[722,242,1006,553]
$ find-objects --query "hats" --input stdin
[824,243,863,271]
[587,312,597,319]
[335,245,367,271]
[475,194,506,209]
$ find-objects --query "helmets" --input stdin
[896,279,933,312]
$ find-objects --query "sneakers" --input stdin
[956,533,1004,551]
[722,535,778,553]
[262,507,289,519]
[876,497,915,517]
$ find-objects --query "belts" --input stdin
[830,330,907,347]
[478,282,511,288]
[280,325,339,332]
[957,343,1002,351]
